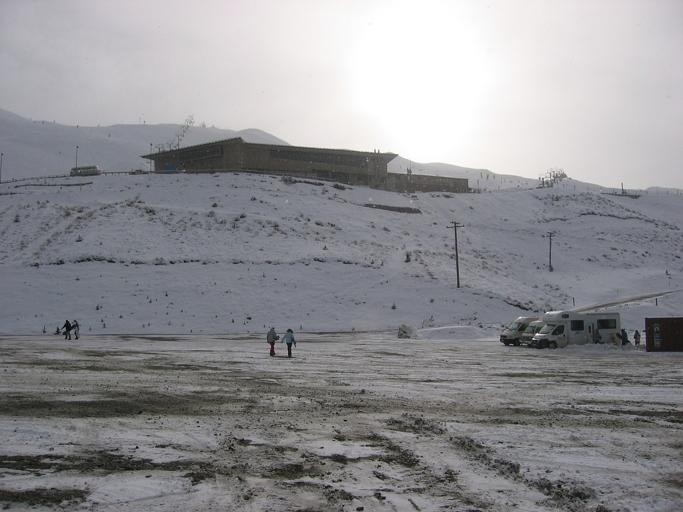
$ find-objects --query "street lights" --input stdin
[76,146,79,168]
[150,143,153,172]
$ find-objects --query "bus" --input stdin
[70,165,99,176]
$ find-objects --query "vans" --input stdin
[500,310,621,349]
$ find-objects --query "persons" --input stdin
[621,328,628,345]
[280,329,296,358]
[633,329,640,347]
[266,327,279,356]
[54,319,80,340]
[594,328,605,344]
